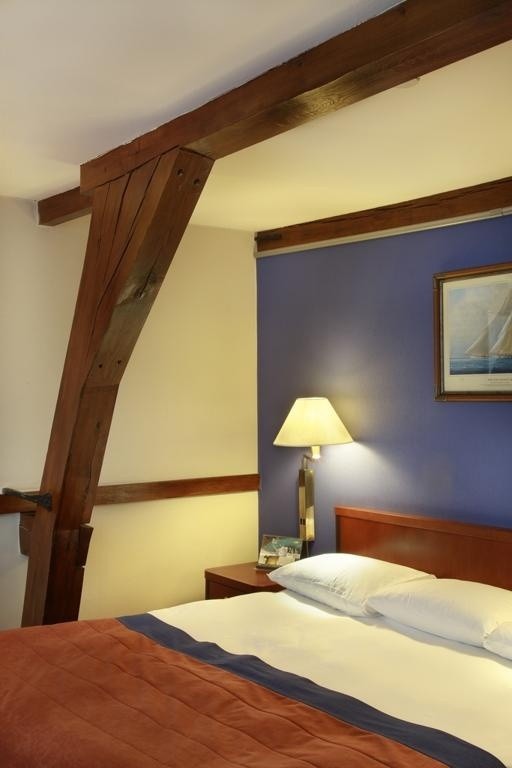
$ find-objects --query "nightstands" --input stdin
[203,559,285,600]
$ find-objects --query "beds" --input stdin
[1,505,512,768]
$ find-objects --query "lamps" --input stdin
[271,395,354,543]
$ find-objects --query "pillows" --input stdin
[264,550,512,660]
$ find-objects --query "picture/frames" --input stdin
[433,261,512,402]
